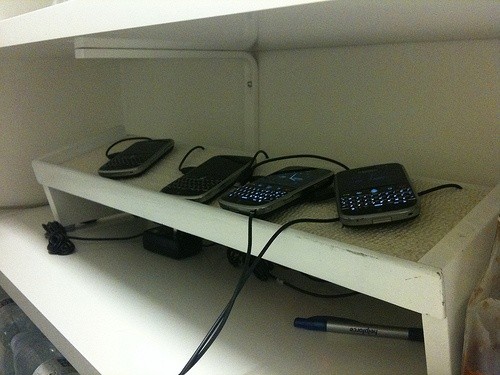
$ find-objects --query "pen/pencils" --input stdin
[293,315,424,343]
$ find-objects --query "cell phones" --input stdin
[99,138,175,179]
[161,154,256,203]
[334,162,421,227]
[218,165,335,216]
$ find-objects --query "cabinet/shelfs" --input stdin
[0,2,500,375]
